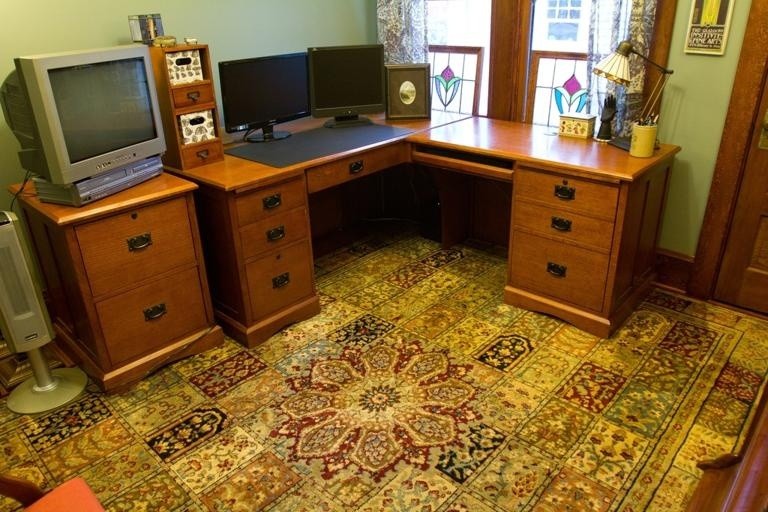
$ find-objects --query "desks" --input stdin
[165,110,683,347]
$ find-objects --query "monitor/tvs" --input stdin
[0,43,170,208]
[217,52,312,144]
[307,43,387,129]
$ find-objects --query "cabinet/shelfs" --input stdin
[10,173,226,395]
[503,158,673,338]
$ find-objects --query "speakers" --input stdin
[0,210,87,415]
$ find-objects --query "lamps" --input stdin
[589,40,674,151]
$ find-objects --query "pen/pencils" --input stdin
[640,112,659,126]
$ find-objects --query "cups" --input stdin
[630,122,657,157]
